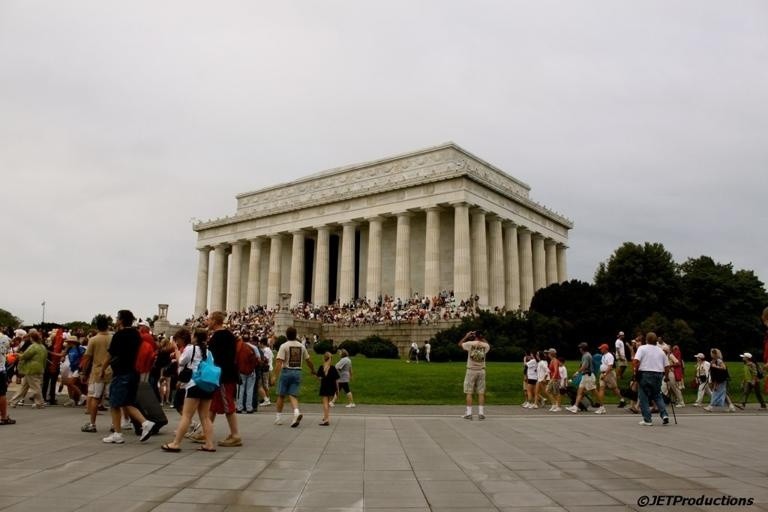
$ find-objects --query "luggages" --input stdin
[559,385,590,412]
[129,381,169,436]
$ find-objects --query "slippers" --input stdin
[196,446,216,453]
[218,435,243,447]
[161,443,182,452]
[191,435,206,443]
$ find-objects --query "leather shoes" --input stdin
[319,421,329,426]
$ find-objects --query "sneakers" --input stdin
[139,420,156,442]
[81,421,123,444]
[522,400,768,426]
[327,402,356,409]
[479,414,486,419]
[462,414,473,419]
[273,413,303,428]
[7,394,271,414]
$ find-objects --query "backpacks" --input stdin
[750,361,764,380]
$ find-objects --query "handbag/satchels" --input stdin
[134,330,159,375]
[178,345,196,384]
[592,354,602,373]
[192,350,222,393]
[710,358,732,385]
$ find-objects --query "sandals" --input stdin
[0,416,16,425]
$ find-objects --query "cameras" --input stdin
[471,332,475,336]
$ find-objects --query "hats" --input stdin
[64,336,81,344]
[542,331,753,360]
